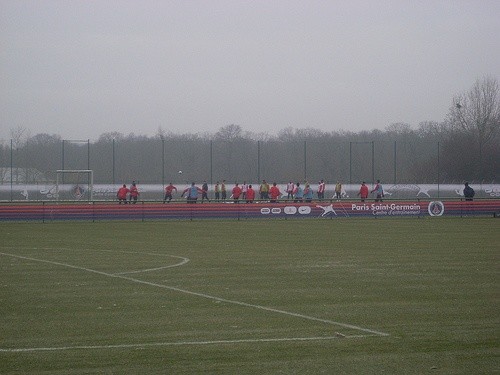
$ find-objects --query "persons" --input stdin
[118,182,139,204]
[371,179,384,203]
[181,182,207,204]
[359,182,369,202]
[222,179,226,203]
[162,182,177,204]
[286,179,313,203]
[73,184,85,200]
[335,180,341,201]
[269,182,280,203]
[259,180,270,199]
[201,183,211,203]
[232,180,255,203]
[214,181,219,202]
[463,182,475,216]
[316,178,325,201]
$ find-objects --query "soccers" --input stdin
[177,171,183,175]
